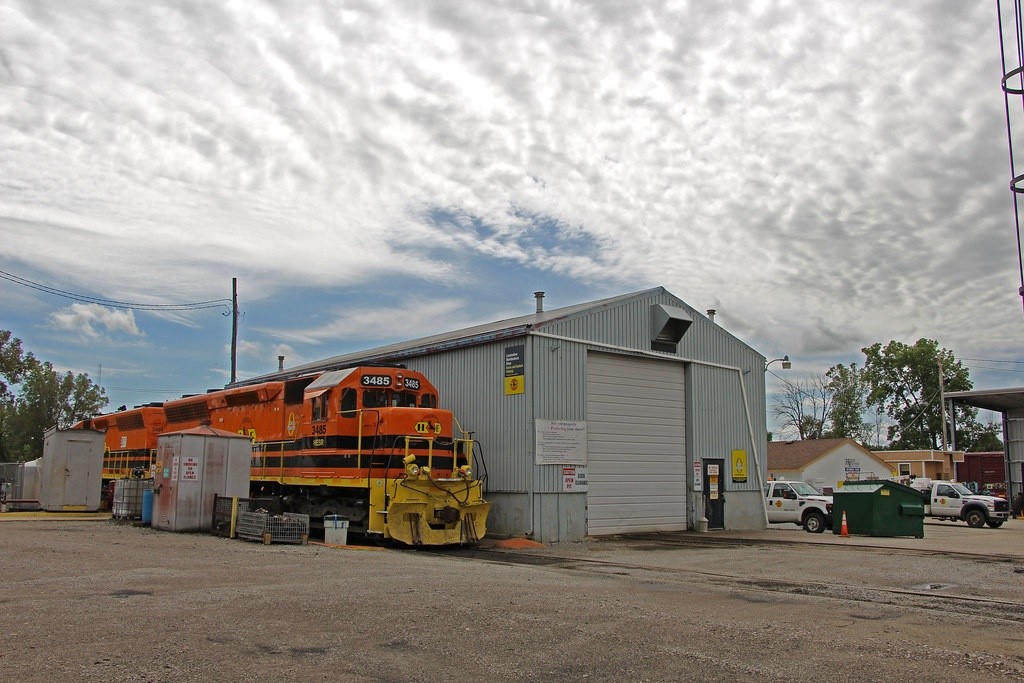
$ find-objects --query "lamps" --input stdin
[763,355,792,372]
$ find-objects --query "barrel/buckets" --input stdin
[141,489,153,523]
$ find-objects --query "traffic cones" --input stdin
[838,510,850,538]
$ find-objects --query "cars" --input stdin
[765,481,833,533]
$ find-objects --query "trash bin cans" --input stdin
[833,479,925,539]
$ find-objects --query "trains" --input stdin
[69,364,491,549]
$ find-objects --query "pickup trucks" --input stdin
[924,482,1009,528]
